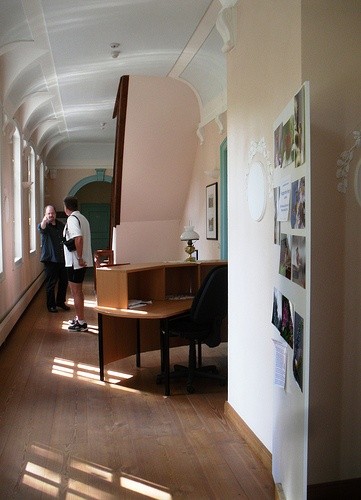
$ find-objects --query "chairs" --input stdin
[155,264,228,392]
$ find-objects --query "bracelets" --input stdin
[78,256,83,259]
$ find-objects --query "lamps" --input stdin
[179,225,200,262]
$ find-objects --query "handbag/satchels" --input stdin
[63,238,76,251]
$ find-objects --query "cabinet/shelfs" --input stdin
[95,260,229,382]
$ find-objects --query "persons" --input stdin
[294,177,305,229]
[37,206,72,312]
[62,196,93,332]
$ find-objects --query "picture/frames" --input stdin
[205,182,217,240]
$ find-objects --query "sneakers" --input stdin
[69,319,76,326]
[68,321,88,332]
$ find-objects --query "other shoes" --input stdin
[47,306,58,312]
[57,302,70,310]
